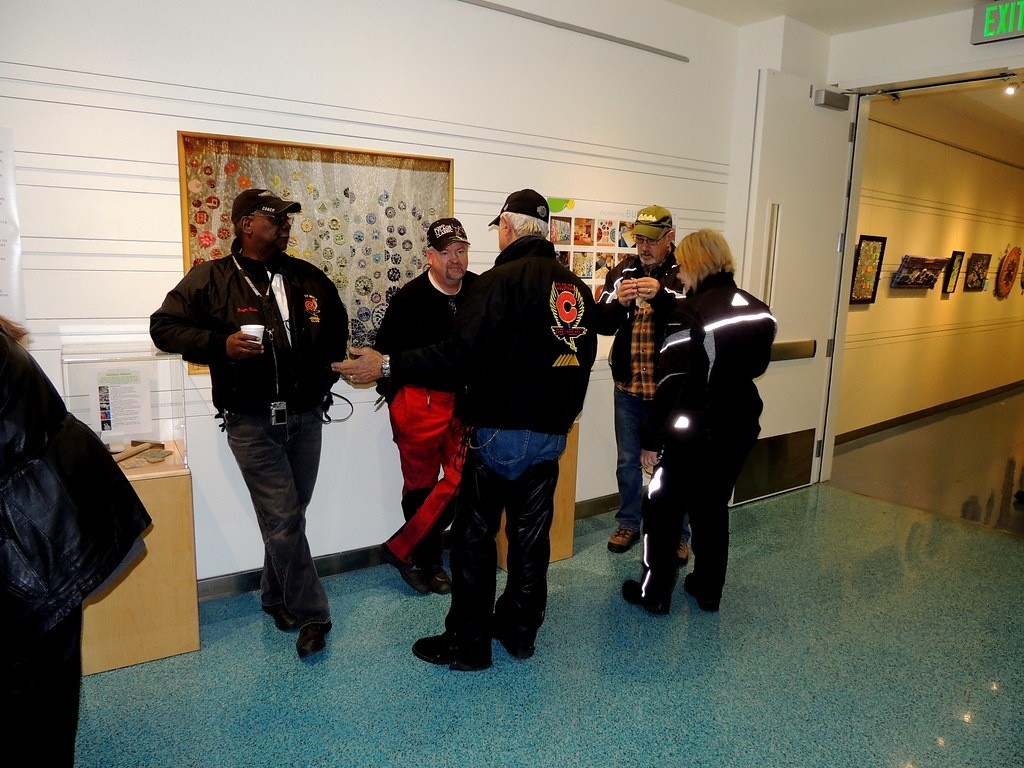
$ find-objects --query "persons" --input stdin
[331,190,776,670]
[0,316,153,767]
[149,188,350,656]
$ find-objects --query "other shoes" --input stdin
[412,633,494,667]
[493,612,535,660]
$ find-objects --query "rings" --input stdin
[350,374,354,380]
[647,288,651,293]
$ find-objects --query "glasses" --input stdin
[245,214,295,226]
[632,230,671,245]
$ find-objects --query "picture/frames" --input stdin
[176,129,454,374]
[850,235,889,305]
[943,251,965,293]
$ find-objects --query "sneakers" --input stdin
[607,525,640,553]
[677,541,689,566]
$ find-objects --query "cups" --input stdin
[241,324,265,345]
[632,278,650,308]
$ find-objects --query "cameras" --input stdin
[270,402,288,426]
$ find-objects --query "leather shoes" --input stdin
[622,580,669,614]
[296,622,332,657]
[261,603,298,632]
[685,572,721,613]
[379,543,451,597]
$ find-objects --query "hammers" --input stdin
[112,440,165,462]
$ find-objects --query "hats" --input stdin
[489,189,549,227]
[630,205,672,240]
[231,189,302,224]
[426,218,471,252]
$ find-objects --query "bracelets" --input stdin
[382,354,392,378]
